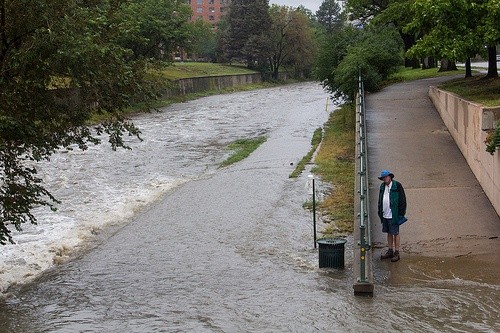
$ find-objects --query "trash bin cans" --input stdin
[316,236,347,270]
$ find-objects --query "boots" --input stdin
[391,250,400,262]
[381,247,394,259]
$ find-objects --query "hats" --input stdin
[378,169,395,181]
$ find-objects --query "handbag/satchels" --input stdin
[397,215,407,225]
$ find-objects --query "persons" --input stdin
[378,170,408,262]
